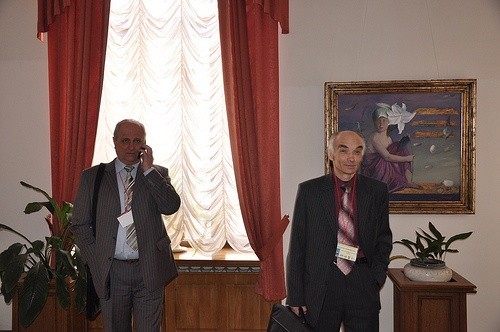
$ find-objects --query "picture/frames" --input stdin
[324,79,477,216]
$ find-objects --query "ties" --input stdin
[123,165,139,252]
[336,184,356,276]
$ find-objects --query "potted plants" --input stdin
[390,222,474,282]
[0,181,89,328]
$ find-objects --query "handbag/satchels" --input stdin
[85,264,102,322]
[267,303,313,332]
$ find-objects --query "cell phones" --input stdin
[138,148,146,158]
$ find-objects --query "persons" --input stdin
[72,119,181,331]
[286,130,393,332]
